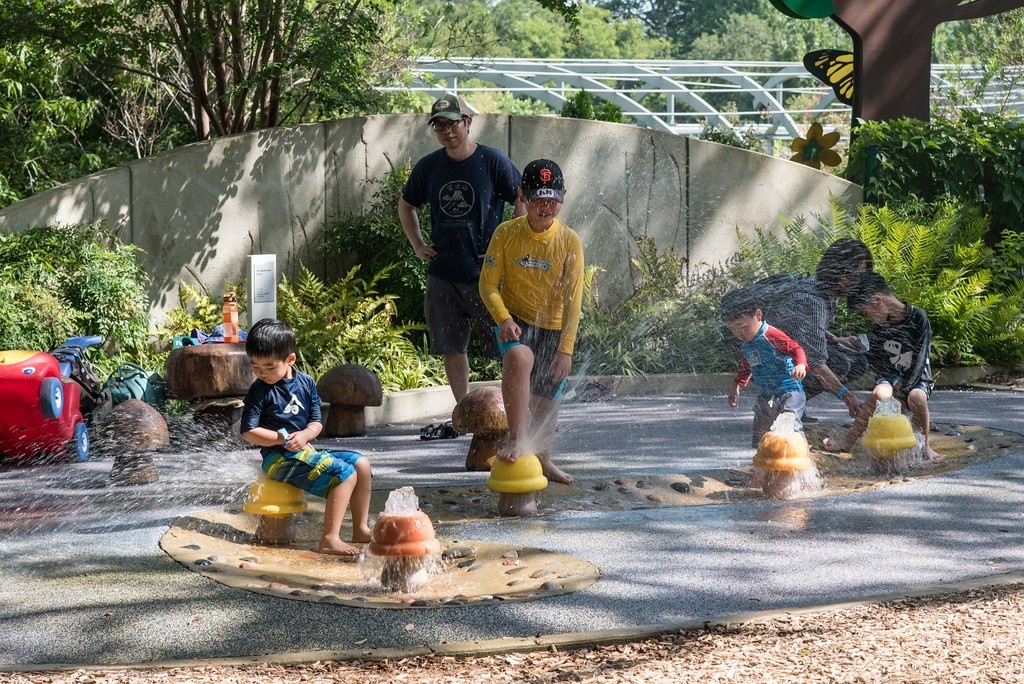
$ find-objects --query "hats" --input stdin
[521,158,565,204]
[428,94,471,126]
[192,322,248,344]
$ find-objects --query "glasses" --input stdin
[431,118,465,132]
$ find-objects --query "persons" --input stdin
[721,238,874,449]
[239,318,375,556]
[478,158,585,484]
[399,93,527,441]
[822,271,943,461]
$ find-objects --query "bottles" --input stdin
[222,291,239,343]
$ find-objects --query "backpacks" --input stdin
[106,362,166,412]
[722,271,826,353]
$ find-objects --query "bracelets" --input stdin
[836,386,847,399]
[893,376,901,387]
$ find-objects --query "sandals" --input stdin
[420,421,458,441]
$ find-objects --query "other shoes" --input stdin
[801,411,819,425]
